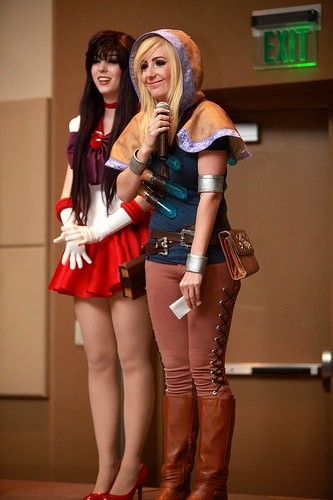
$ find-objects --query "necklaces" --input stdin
[104,101,117,110]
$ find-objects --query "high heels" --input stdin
[103,462,147,500]
[84,457,122,499]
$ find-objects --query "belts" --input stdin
[142,225,219,255]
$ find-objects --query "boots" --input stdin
[156,395,193,498]
[189,399,236,498]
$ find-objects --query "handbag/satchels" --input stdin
[217,228,261,281]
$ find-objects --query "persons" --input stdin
[105,29,252,500]
[48,30,159,500]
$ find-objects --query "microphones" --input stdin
[155,101,170,160]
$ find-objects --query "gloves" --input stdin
[53,197,92,270]
[60,198,144,248]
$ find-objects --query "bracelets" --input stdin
[185,254,207,274]
[129,149,148,175]
